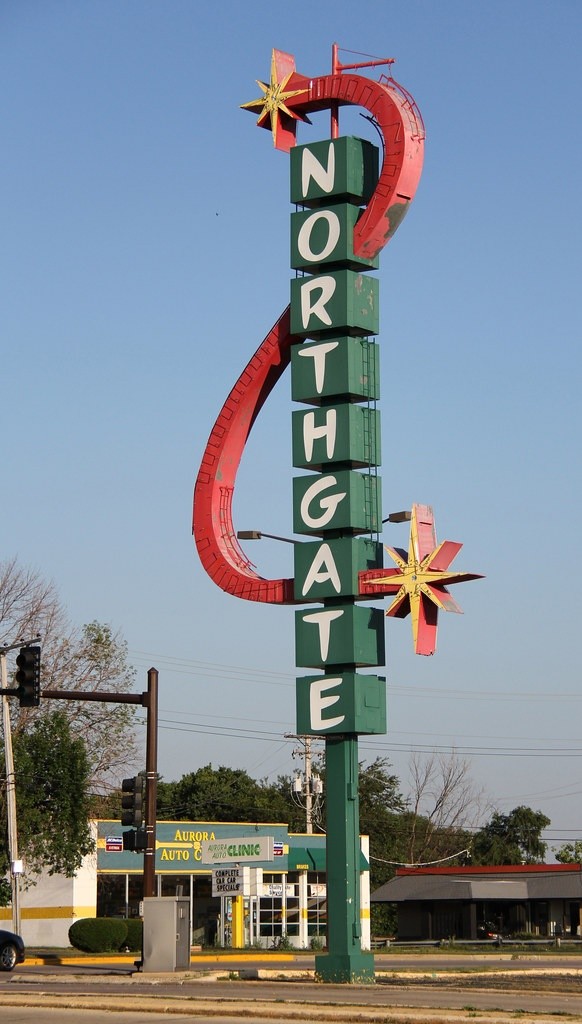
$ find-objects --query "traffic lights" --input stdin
[121,778,134,826]
[16,651,27,700]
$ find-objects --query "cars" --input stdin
[0,930,26,970]
[444,911,519,941]
